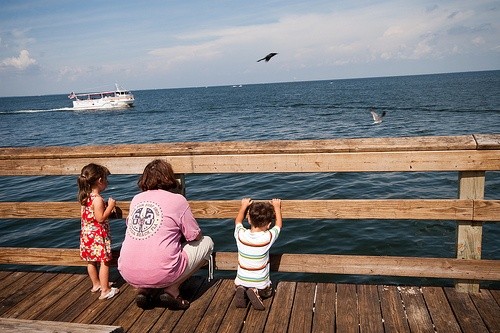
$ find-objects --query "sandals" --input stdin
[235,286,246,308]
[246,289,265,310]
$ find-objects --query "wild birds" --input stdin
[370,109,387,124]
[256,52,278,63]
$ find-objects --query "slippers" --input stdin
[99,287,119,300]
[91,281,113,293]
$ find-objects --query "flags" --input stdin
[68,92,76,99]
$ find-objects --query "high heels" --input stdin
[136,292,154,305]
[160,292,190,308]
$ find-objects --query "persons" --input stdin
[77,163,120,300]
[233,197,283,310]
[118,159,214,309]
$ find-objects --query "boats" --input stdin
[68,83,136,111]
